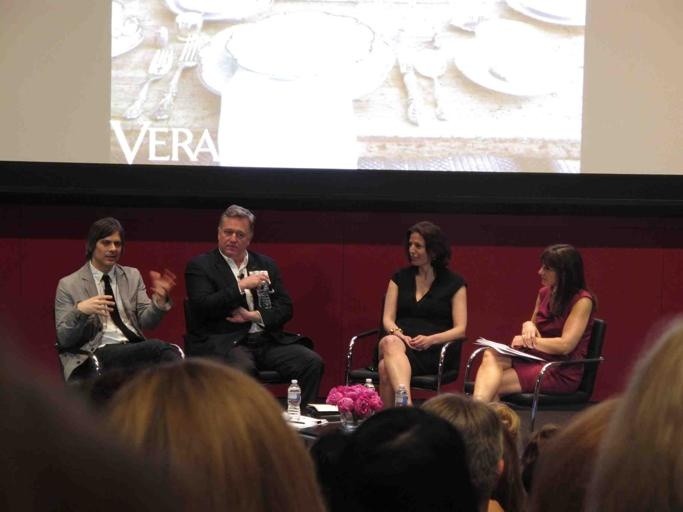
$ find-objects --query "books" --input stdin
[472,334,547,364]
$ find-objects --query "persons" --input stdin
[311,431,353,490]
[376,220,467,406]
[469,243,597,409]
[329,408,483,512]
[421,312,683,512]
[52,218,184,385]
[2,371,191,512]
[104,357,326,512]
[179,202,325,407]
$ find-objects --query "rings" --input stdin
[521,334,525,338]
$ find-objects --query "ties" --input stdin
[102,276,140,342]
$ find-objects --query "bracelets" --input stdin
[385,327,402,335]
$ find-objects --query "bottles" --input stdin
[286,379,301,423]
[363,377,375,390]
[254,280,272,311]
[393,384,409,408]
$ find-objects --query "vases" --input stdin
[340,411,367,433]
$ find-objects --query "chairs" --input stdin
[463,318,606,432]
[182,297,287,384]
[343,294,458,396]
[54,342,186,379]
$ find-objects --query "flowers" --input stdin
[326,384,384,416]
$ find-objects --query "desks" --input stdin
[279,398,364,442]
